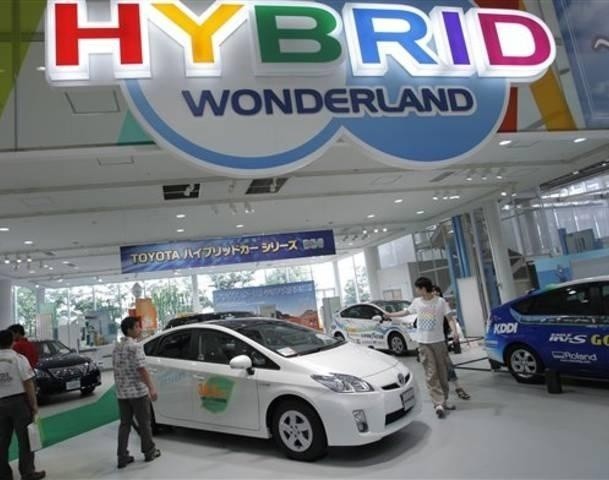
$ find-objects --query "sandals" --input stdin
[455,389,470,399]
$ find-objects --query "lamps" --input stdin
[342,164,521,243]
[242,197,254,216]
[2,251,44,271]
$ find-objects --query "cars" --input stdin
[160,311,261,330]
[26,335,103,406]
[484,275,609,386]
[327,297,464,358]
[129,314,424,462]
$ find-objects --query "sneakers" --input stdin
[117,456,134,469]
[435,404,456,418]
[21,470,45,480]
[144,449,160,462]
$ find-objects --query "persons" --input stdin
[0,330,45,480]
[8,324,38,396]
[382,276,460,416]
[112,317,162,468]
[413,285,470,400]
